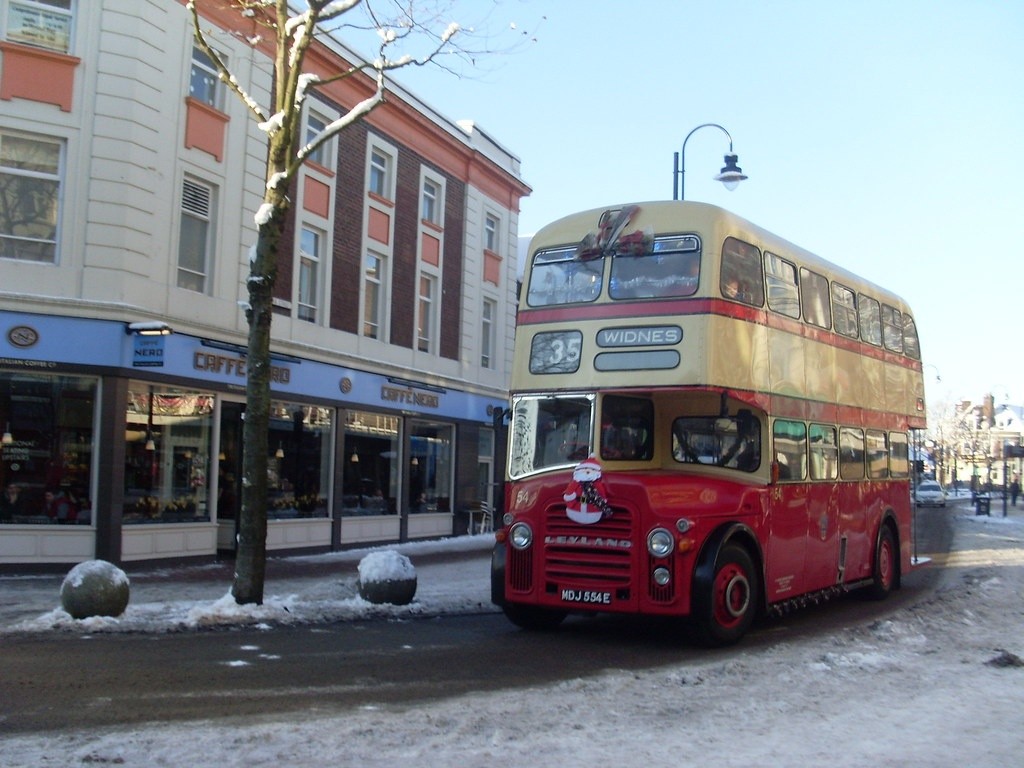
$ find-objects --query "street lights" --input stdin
[674,122,749,200]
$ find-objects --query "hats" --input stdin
[1014,475,1018,480]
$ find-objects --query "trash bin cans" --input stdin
[975,497,992,517]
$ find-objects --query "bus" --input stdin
[152,448,204,492]
[44,427,144,497]
[491,197,930,645]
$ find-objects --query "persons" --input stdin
[969,475,982,506]
[1009,479,1023,506]
[724,278,738,298]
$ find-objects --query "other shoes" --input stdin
[1011,503,1016,506]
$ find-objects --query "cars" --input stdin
[914,480,947,508]
